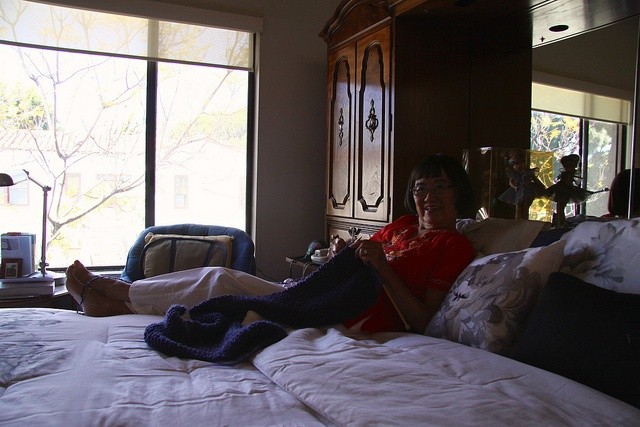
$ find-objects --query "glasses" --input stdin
[410,182,454,197]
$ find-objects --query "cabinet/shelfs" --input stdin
[322,219,385,255]
[317,0,469,227]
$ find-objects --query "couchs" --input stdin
[118,222,257,287]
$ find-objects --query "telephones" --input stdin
[301,248,328,279]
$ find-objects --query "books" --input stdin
[1,232,36,275]
[0,278,56,299]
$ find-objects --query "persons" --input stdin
[546,154,593,219]
[600,167,640,219]
[65,155,480,337]
[497,148,546,220]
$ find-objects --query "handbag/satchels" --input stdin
[304,240,327,261]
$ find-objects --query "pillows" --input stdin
[453,217,546,258]
[555,217,640,295]
[427,239,566,353]
[139,230,233,276]
[505,268,639,404]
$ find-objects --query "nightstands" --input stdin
[284,248,331,283]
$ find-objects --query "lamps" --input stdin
[0,168,68,287]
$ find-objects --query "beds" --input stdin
[0,217,640,424]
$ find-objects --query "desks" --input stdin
[0,282,76,309]
